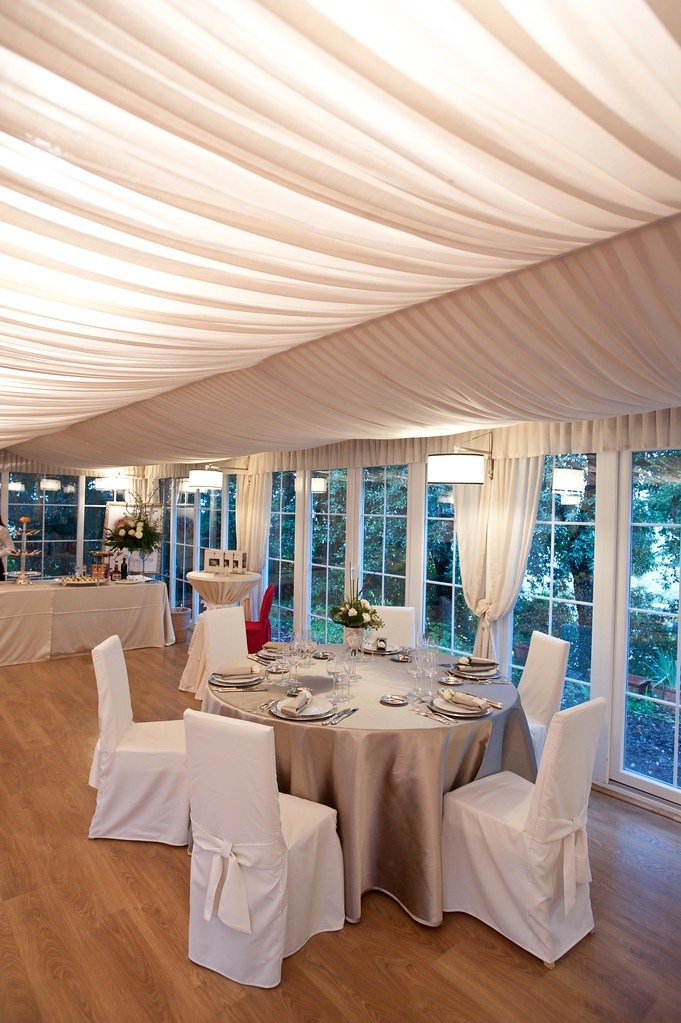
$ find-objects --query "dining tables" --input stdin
[0,575,175,667]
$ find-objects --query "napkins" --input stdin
[377,637,388,650]
[262,641,298,652]
[281,693,309,717]
[456,657,500,672]
[212,666,261,680]
[452,691,491,713]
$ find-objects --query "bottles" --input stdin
[111,560,122,581]
[121,557,128,579]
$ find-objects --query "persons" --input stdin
[0,515,14,582]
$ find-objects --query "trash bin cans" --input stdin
[170,607,191,643]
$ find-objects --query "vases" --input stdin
[128,550,141,574]
[345,627,365,650]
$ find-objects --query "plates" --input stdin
[312,650,335,659]
[210,672,266,687]
[270,695,336,721]
[447,663,501,680]
[390,654,414,662]
[439,676,465,685]
[287,686,313,697]
[358,640,403,655]
[380,694,410,705]
[257,649,287,660]
[117,579,141,585]
[428,697,493,717]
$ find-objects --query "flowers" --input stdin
[301,690,312,705]
[443,689,454,700]
[251,665,260,674]
[459,657,469,664]
[328,561,385,631]
[97,478,171,563]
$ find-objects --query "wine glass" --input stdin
[265,632,439,704]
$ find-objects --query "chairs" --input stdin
[246,584,275,654]
[88,605,606,988]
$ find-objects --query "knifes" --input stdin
[321,706,359,725]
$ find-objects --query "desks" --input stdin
[179,569,262,698]
[201,643,538,927]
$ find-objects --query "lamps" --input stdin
[179,479,208,494]
[427,432,494,484]
[294,478,327,493]
[552,468,584,505]
[8,480,76,493]
[189,456,250,490]
[95,477,129,491]
